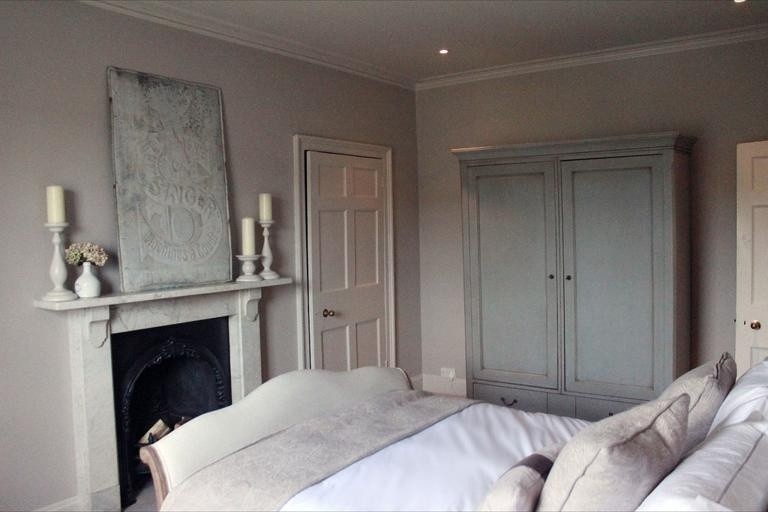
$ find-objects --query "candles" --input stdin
[46,184,67,224]
[241,216,256,256]
[258,192,272,221]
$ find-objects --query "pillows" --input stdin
[475,440,567,512]
[704,353,768,437]
[536,392,692,512]
[636,420,768,511]
[658,352,738,463]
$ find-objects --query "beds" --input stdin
[139,364,590,512]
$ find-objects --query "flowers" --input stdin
[64,241,109,268]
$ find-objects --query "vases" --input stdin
[74,262,102,299]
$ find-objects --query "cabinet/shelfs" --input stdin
[450,130,697,421]
